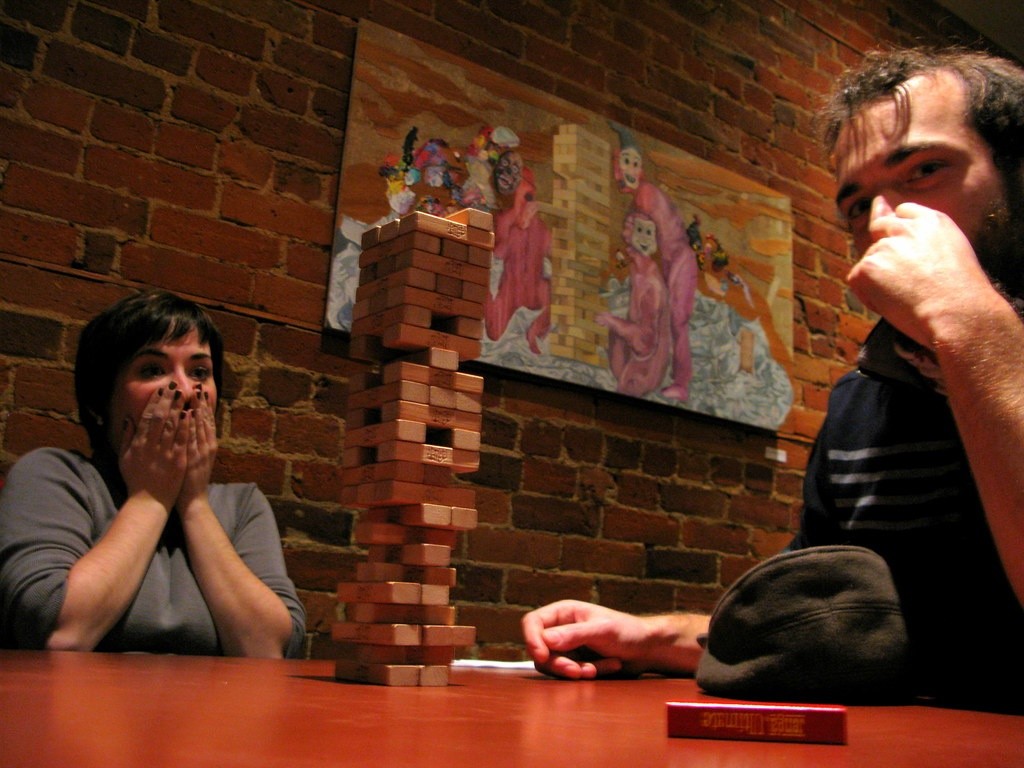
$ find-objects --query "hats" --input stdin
[697,545,939,705]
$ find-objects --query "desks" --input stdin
[0,647,1024,768]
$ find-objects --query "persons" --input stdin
[0,288,308,660]
[521,33,1024,696]
